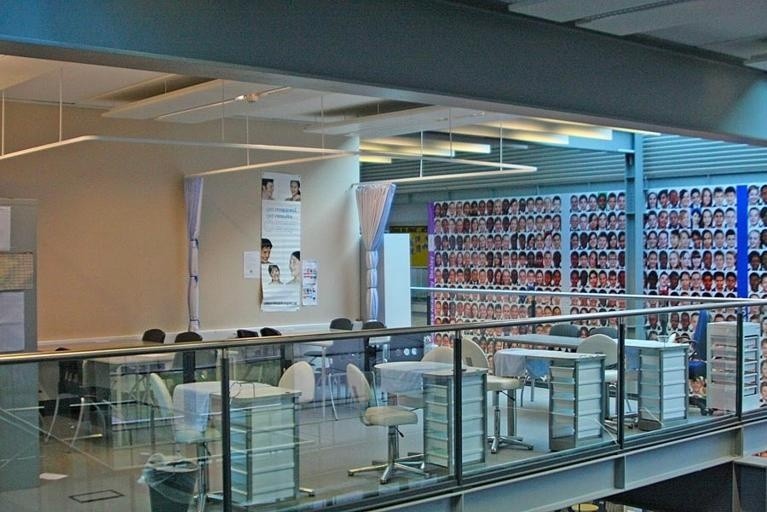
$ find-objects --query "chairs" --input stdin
[588,327,620,339]
[150,370,221,511]
[420,347,454,365]
[344,362,430,488]
[462,336,533,456]
[688,306,713,415]
[575,333,638,430]
[552,326,581,338]
[43,314,390,455]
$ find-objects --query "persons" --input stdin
[643,291,766,410]
[434,285,562,383]
[260,238,274,264]
[642,185,767,293]
[261,178,274,200]
[433,194,562,287]
[570,289,626,396]
[267,264,284,285]
[284,250,301,285]
[569,193,626,289]
[284,180,301,201]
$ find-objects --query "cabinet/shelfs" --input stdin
[705,320,761,417]
[0,195,41,492]
[359,232,413,329]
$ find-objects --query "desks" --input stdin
[171,378,303,511]
[375,358,491,478]
[490,333,693,432]
[492,347,606,450]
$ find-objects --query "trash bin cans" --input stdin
[146,456,199,512]
